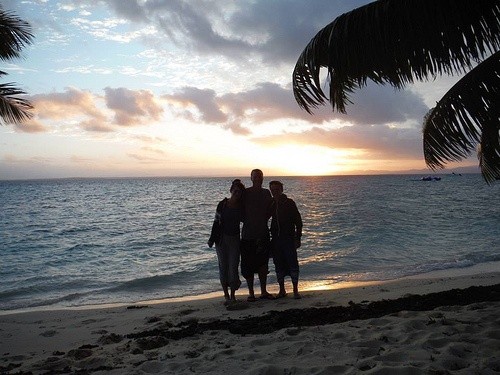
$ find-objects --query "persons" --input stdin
[267,181,303,299]
[235,168,274,302]
[208,179,246,305]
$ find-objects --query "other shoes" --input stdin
[259,293,275,300]
[293,293,301,299]
[223,294,237,305]
[274,292,288,300]
[246,295,255,302]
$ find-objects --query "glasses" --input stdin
[271,188,281,191]
